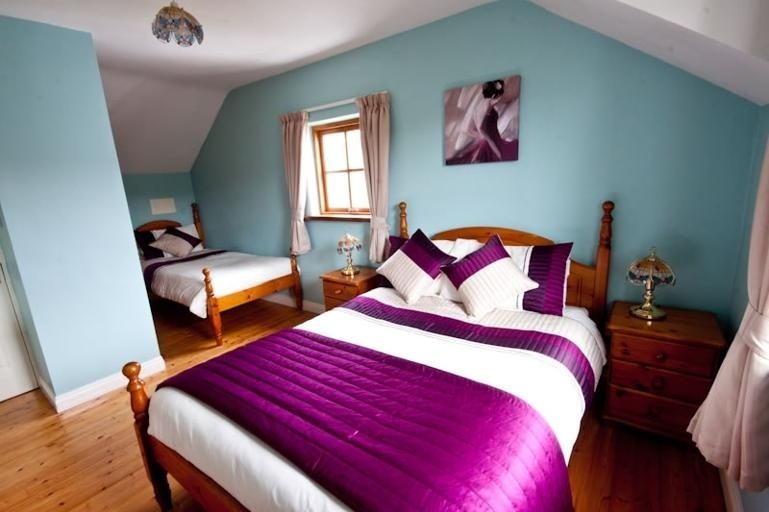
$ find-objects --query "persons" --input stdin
[446,80,518,164]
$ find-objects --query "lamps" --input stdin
[151,0,203,48]
[626,246,676,320]
[337,233,362,275]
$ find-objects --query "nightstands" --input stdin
[602,300,728,451]
[320,266,386,311]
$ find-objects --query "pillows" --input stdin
[135,224,203,261]
[386,235,476,297]
[440,238,573,316]
[440,233,540,318]
[149,226,203,258]
[376,228,457,304]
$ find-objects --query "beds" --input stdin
[134,203,305,346]
[122,201,614,512]
